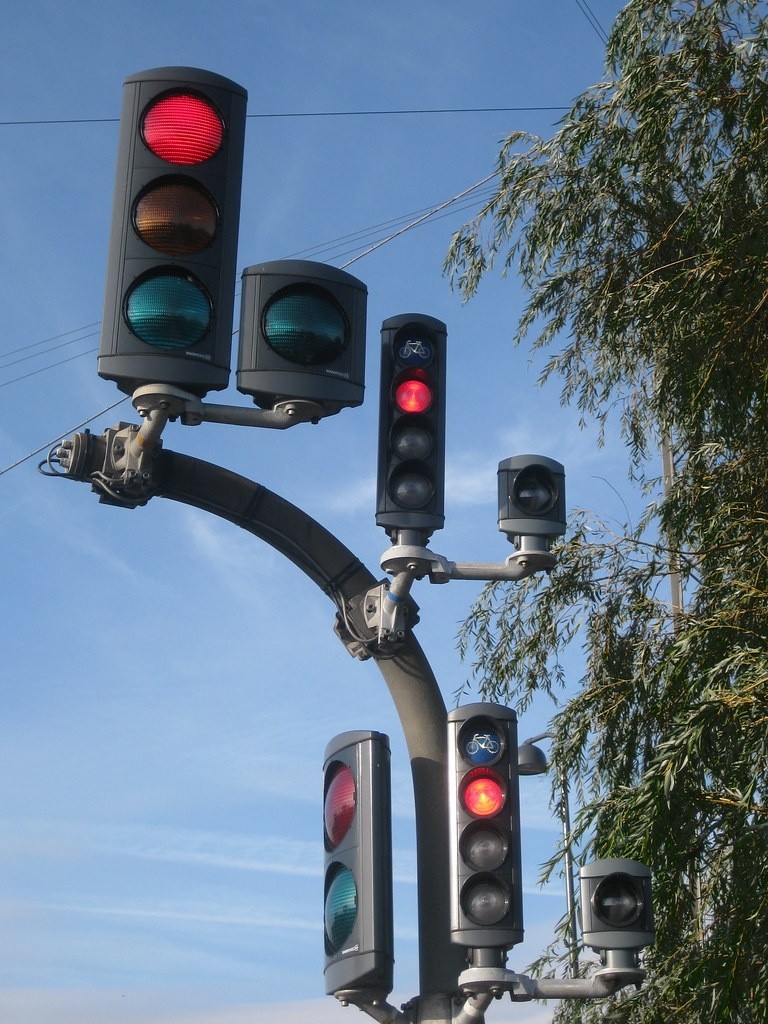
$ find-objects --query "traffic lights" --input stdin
[497,454,568,536]
[580,858,656,949]
[374,312,449,534]
[321,729,394,995]
[447,701,525,947]
[95,64,249,396]
[237,258,368,404]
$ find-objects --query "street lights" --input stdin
[517,730,580,980]
